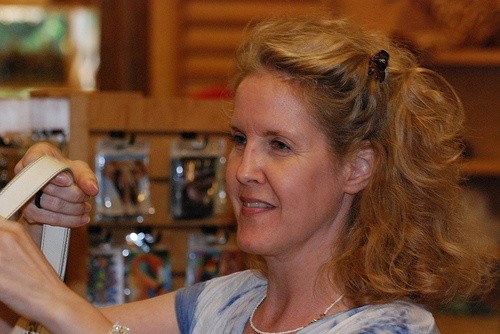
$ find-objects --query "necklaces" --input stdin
[250,293,345,334]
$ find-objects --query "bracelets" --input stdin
[108,321,130,334]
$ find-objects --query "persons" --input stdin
[0,15,500,334]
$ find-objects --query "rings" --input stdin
[34,190,44,208]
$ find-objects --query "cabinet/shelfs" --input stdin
[28,92,253,306]
[389,33,498,178]
